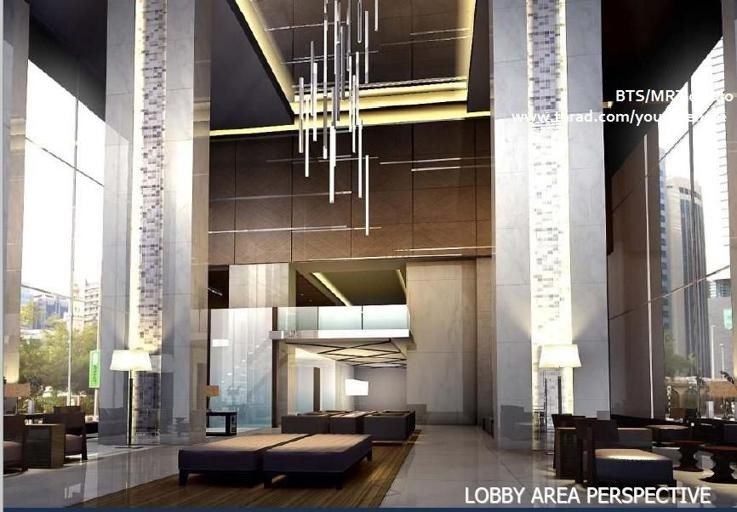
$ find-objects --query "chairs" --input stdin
[4,416,29,473]
[41,410,89,462]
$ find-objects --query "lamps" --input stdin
[538,344,581,456]
[289,1,381,239]
[204,385,220,413]
[3,382,32,417]
[108,346,152,449]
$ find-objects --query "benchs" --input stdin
[263,433,373,490]
[177,433,308,488]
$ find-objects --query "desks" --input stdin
[206,412,238,436]
[24,422,67,470]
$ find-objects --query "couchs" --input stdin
[280,407,418,443]
[546,413,737,498]
